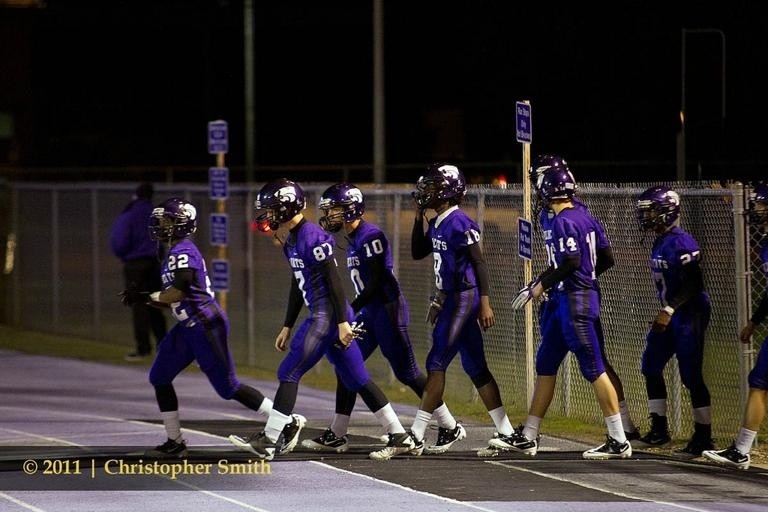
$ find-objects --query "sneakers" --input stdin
[144,437,190,462]
[672,439,716,458]
[368,431,416,464]
[380,428,425,456]
[122,348,151,364]
[477,434,521,457]
[489,428,540,457]
[229,429,277,461]
[583,434,633,460]
[278,413,306,455]
[299,427,351,456]
[624,427,672,449]
[426,423,467,456]
[703,446,751,471]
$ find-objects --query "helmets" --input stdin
[539,170,580,199]
[410,161,468,209]
[743,184,768,228]
[255,178,306,230]
[633,186,676,228]
[528,154,567,190]
[149,198,197,243]
[319,182,367,233]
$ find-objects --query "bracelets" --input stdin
[662,305,676,315]
[150,291,163,304]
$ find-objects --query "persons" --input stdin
[628,185,720,461]
[527,154,643,445]
[486,163,634,462]
[700,180,767,473]
[300,180,469,456]
[379,160,518,459]
[114,196,309,460]
[110,180,171,361]
[225,177,417,463]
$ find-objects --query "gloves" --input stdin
[511,288,534,312]
[117,277,152,307]
[425,289,444,327]
[333,321,366,353]
